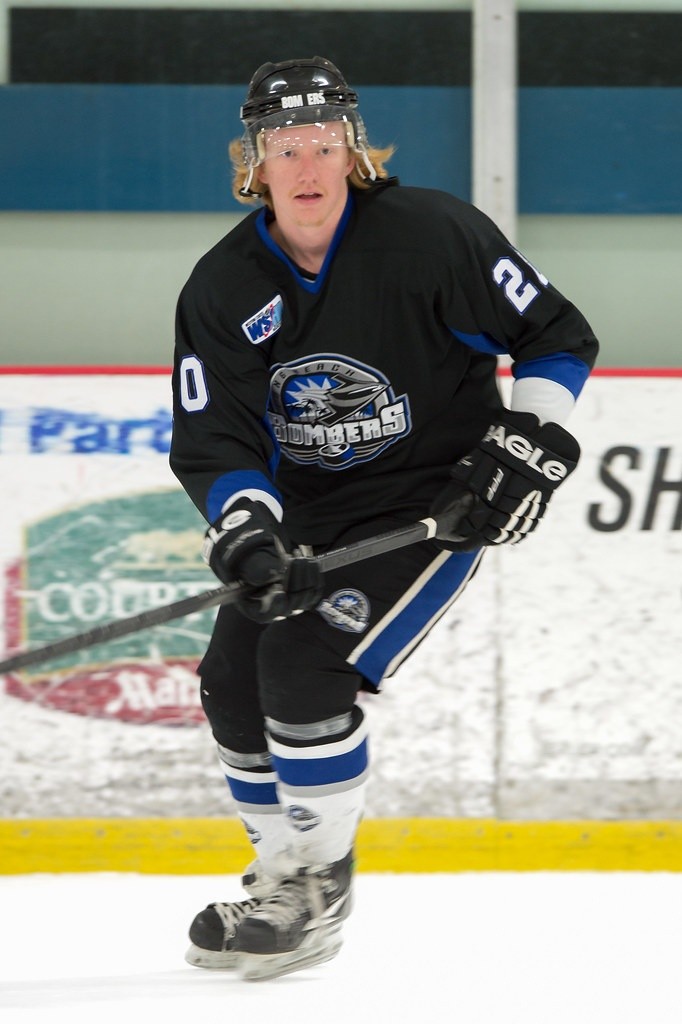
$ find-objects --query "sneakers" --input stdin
[181,858,294,968]
[232,846,355,984]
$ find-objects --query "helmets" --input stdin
[239,55,360,136]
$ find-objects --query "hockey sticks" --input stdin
[0,508,466,678]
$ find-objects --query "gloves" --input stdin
[431,407,580,553]
[201,495,324,624]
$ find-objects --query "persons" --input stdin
[167,54,599,982]
